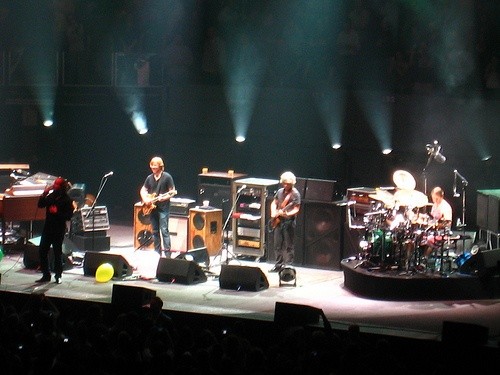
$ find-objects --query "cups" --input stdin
[203,200,209,207]
[202,168,208,174]
[228,170,234,177]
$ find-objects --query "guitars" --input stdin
[142,190,177,216]
[268,199,298,233]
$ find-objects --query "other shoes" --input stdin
[36,275,51,282]
[166,254,171,259]
[268,266,279,272]
[55,274,62,284]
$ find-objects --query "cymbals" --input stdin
[392,170,416,190]
[393,190,428,207]
[369,195,393,201]
[376,188,395,206]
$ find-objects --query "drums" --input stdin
[363,211,451,265]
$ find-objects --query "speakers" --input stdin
[112,284,156,307]
[265,178,347,271]
[476,189,500,233]
[219,265,269,292]
[273,302,321,325]
[23,236,70,270]
[134,183,233,285]
[83,252,133,278]
[458,248,500,276]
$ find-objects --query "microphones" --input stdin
[104,171,114,177]
[237,185,247,193]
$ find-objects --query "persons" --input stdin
[34,178,73,284]
[430,187,452,246]
[140,156,177,258]
[267,172,301,273]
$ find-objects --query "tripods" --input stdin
[354,227,377,270]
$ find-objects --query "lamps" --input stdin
[279,267,297,287]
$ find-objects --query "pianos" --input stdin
[0,171,59,254]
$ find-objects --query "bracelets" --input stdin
[43,192,48,195]
